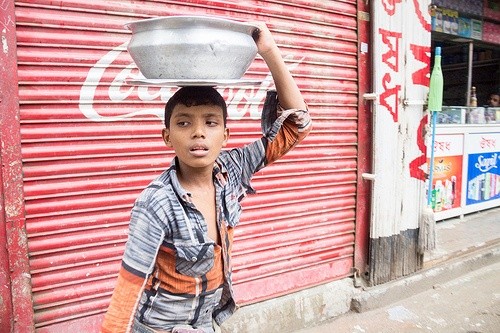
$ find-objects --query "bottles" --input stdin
[470,87,477,107]
[472,173,500,200]
[425,177,456,211]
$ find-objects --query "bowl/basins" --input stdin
[124,15,262,79]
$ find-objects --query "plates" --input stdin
[125,79,263,88]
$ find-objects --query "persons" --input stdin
[487,93,500,107]
[100,21,312,333]
